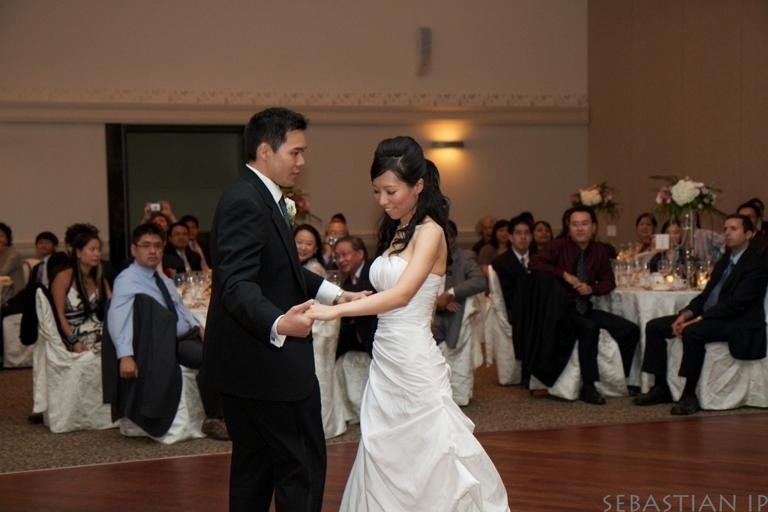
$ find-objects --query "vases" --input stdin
[684,208,699,290]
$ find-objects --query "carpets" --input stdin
[2,352,767,477]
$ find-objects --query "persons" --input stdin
[197,107,373,512]
[304,136,511,512]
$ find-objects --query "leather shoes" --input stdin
[202,417,231,440]
[532,388,548,400]
[626,383,672,406]
[670,394,701,415]
[580,385,606,405]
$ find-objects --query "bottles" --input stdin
[708,246,722,263]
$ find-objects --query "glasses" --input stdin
[133,241,163,248]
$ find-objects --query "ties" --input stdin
[703,260,735,313]
[192,241,206,270]
[576,250,587,316]
[278,194,293,239]
[521,257,525,267]
[153,270,179,323]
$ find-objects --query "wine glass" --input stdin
[613,242,645,260]
[175,270,211,307]
[327,234,340,260]
[611,258,709,289]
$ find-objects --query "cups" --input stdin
[313,269,342,283]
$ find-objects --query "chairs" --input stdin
[1,244,768,446]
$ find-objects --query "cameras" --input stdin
[150,203,161,212]
[329,237,338,245]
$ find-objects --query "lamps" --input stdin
[422,138,466,150]
[280,183,324,230]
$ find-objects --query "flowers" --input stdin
[648,174,731,230]
[569,177,627,224]
